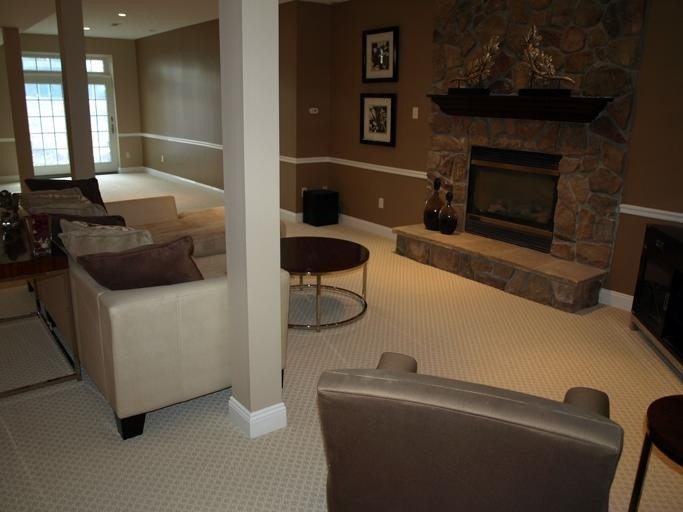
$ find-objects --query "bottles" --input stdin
[437,191,458,235]
[423,178,445,230]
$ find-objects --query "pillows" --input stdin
[21,177,204,293]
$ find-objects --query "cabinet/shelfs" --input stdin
[625,221,682,383]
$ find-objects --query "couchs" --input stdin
[16,174,293,444]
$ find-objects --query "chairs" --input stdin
[312,346,626,512]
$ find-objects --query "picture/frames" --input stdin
[356,91,399,148]
[359,23,399,84]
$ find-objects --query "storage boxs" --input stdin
[300,187,339,229]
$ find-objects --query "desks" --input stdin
[624,391,682,512]
[0,192,85,400]
